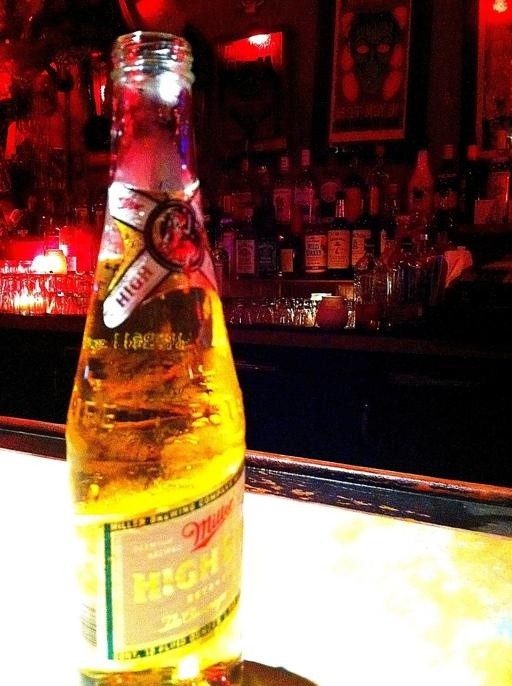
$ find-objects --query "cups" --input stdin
[1,256,93,317]
[221,289,314,328]
[315,296,348,328]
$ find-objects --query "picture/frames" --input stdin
[313,0,432,168]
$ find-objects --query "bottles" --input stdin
[368,141,484,239]
[8,192,108,236]
[352,194,379,275]
[352,218,476,329]
[202,207,298,281]
[206,145,367,223]
[488,128,509,220]
[329,189,352,281]
[299,187,328,277]
[62,29,249,686]
